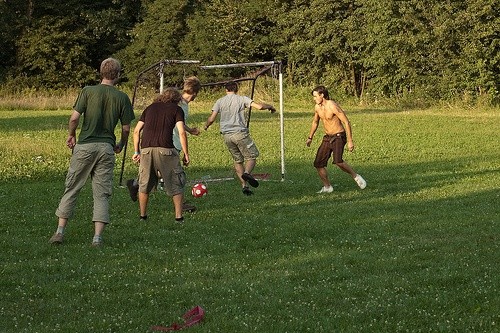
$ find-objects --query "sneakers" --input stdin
[173,202,196,212]
[353,174,367,190]
[315,185,334,193]
[126,179,139,202]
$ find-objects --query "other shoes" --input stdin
[48,233,64,244]
[139,215,147,221]
[175,217,185,223]
[242,187,253,196]
[92,235,104,245]
[242,173,259,188]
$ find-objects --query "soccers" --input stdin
[192,183,208,197]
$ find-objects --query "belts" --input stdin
[323,133,345,143]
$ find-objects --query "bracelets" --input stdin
[135,152,140,154]
[308,137,312,140]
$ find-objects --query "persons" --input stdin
[305,85,367,194]
[204,81,277,196]
[47,57,136,246]
[131,87,190,221]
[126,76,202,212]
